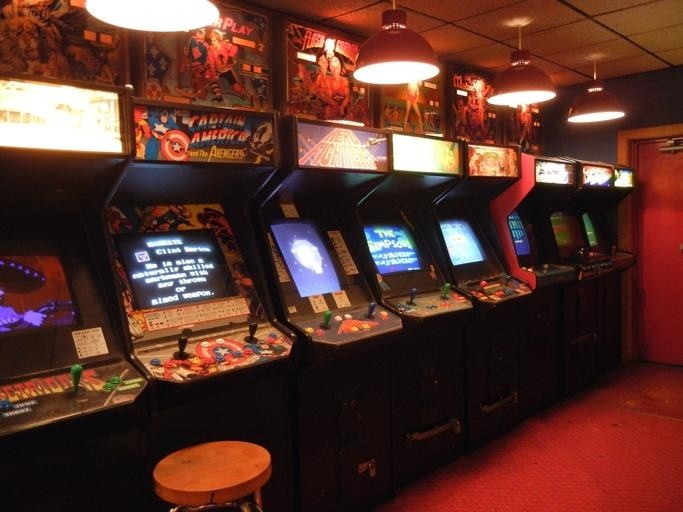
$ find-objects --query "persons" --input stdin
[316,50,351,120]
[404,84,424,135]
[0,287,48,332]
[184,28,253,106]
[145,106,180,160]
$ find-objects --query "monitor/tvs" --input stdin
[360,220,428,282]
[582,210,600,248]
[549,210,578,251]
[265,217,352,299]
[0,248,85,340]
[436,211,494,269]
[114,225,244,311]
[507,209,535,259]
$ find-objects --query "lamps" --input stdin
[485,23,557,106]
[354,0,440,85]
[565,58,627,124]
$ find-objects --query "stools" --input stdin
[153,440,273,512]
